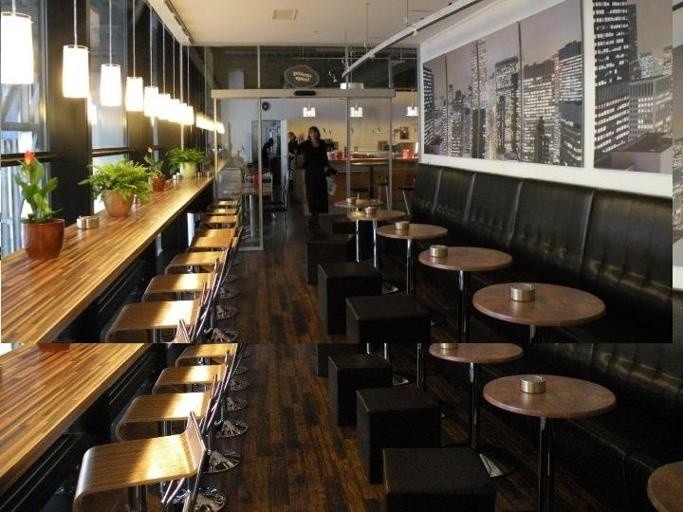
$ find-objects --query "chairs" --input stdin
[264,169,290,237]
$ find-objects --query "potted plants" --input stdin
[78,160,153,218]
[168,148,207,179]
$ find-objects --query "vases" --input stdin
[148,176,167,194]
[23,219,65,259]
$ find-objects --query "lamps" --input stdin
[302,102,315,118]
[338,59,364,90]
[0,0,225,134]
[350,99,363,118]
[407,92,418,116]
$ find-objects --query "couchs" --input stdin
[399,162,672,342]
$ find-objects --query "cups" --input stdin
[335,151,342,160]
[327,152,334,160]
[403,149,409,159]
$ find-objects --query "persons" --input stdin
[299,127,329,213]
[262,139,273,164]
[288,132,298,168]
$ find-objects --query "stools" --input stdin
[72,344,249,512]
[303,196,431,345]
[308,344,498,511]
[99,195,243,343]
[350,181,414,214]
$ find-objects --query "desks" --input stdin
[426,344,683,512]
[351,161,388,197]
[334,195,607,343]
[224,181,272,239]
[1,160,214,344]
[0,345,152,497]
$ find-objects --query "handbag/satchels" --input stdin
[295,154,304,169]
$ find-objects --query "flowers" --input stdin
[144,144,165,176]
[12,151,65,222]
